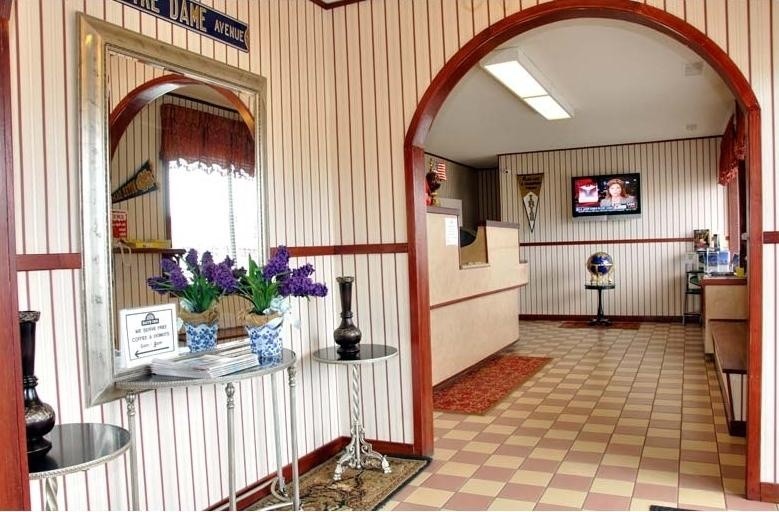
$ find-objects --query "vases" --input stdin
[239,306,284,361]
[181,319,218,354]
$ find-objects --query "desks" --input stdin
[311,343,399,482]
[115,347,304,511]
[29,421,138,512]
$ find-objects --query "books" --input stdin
[685,228,731,290]
[150,352,261,379]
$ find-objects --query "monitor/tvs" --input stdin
[571,173,642,221]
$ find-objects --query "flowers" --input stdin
[235,251,329,316]
[145,247,235,310]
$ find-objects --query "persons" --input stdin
[600,177,635,207]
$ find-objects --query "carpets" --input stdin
[243,450,434,512]
[434,356,554,418]
[556,317,643,331]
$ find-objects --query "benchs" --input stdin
[707,318,749,438]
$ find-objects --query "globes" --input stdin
[586,251,614,285]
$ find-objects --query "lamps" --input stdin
[478,46,575,121]
[335,276,362,357]
[16,310,56,456]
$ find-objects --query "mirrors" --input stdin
[71,9,273,410]
